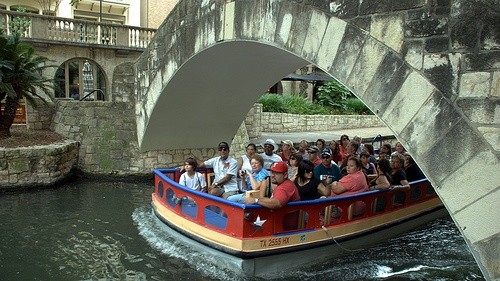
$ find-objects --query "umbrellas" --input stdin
[82,60,94,101]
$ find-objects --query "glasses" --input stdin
[218,147,227,152]
[306,168,314,173]
[321,155,331,159]
[343,137,348,140]
[308,151,315,154]
[289,157,296,160]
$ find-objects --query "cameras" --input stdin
[241,170,246,175]
[320,175,327,180]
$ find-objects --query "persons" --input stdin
[245,162,300,209]
[260,139,283,171]
[238,143,256,175]
[388,154,425,191]
[314,148,341,184]
[179,158,207,192]
[364,144,376,163]
[279,140,296,162]
[316,135,364,167]
[292,160,332,200]
[178,142,239,200]
[331,158,368,197]
[359,152,378,180]
[306,146,322,166]
[285,154,303,179]
[298,139,309,161]
[369,158,391,190]
[379,142,406,160]
[340,140,359,170]
[228,153,270,203]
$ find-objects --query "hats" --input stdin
[306,146,320,153]
[266,162,288,173]
[219,142,229,148]
[259,139,279,153]
[320,147,332,156]
[281,140,293,148]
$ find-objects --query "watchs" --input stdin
[254,197,259,204]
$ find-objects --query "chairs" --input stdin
[207,173,215,188]
